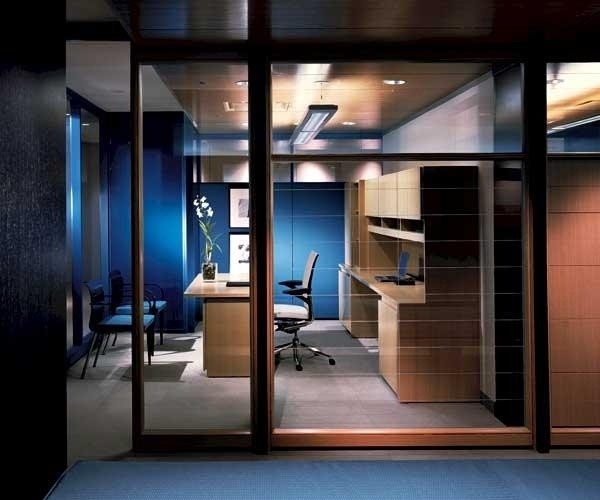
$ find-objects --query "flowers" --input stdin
[192,192,229,265]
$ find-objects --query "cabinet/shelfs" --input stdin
[348,179,401,271]
[364,165,479,302]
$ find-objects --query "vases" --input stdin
[200,261,219,283]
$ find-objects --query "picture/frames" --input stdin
[228,232,251,273]
[228,187,251,230]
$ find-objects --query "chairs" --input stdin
[75,277,153,382]
[101,268,169,357]
[272,250,337,373]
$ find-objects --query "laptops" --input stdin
[376,252,412,281]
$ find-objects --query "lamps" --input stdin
[287,80,340,147]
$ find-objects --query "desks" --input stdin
[336,263,484,406]
[182,271,251,380]
